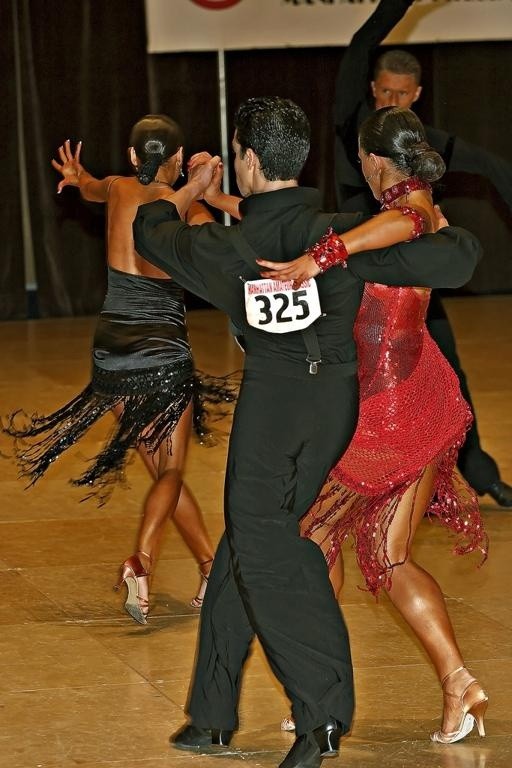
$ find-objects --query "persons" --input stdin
[50,116,223,634]
[130,93,485,766]
[329,0,511,524]
[187,102,490,745]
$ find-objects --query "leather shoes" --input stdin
[487,480,512,509]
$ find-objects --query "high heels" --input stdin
[189,558,213,608]
[281,715,296,730]
[175,723,233,749]
[112,550,153,625]
[278,719,343,768]
[429,664,490,744]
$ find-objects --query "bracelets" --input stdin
[307,225,349,274]
